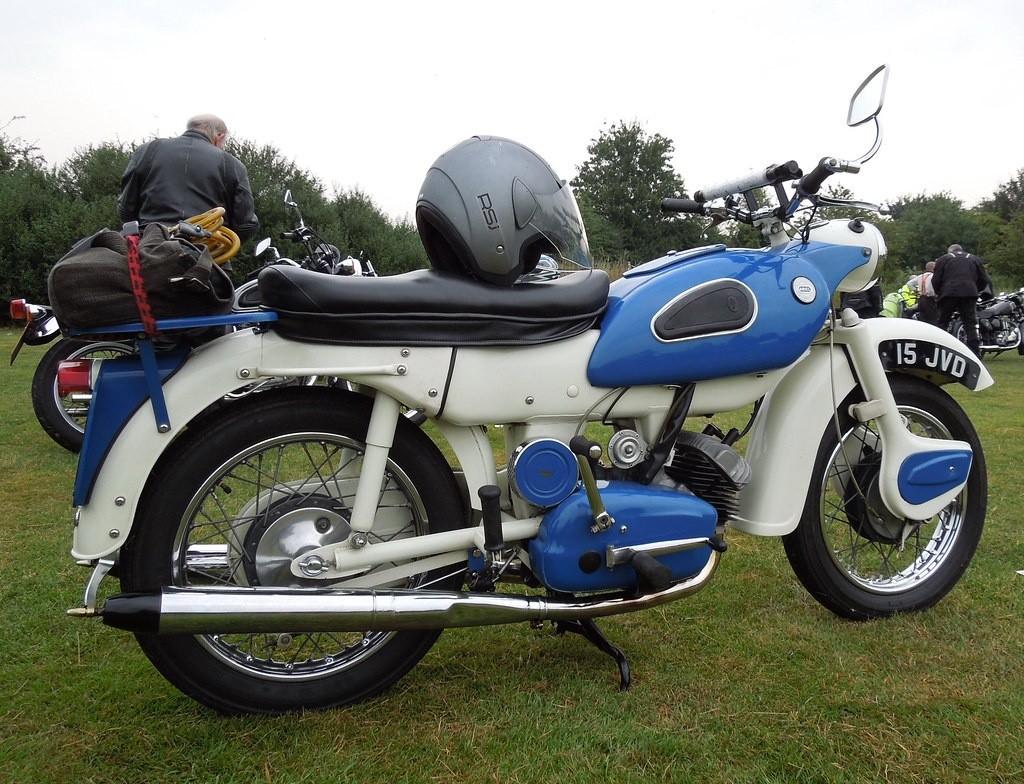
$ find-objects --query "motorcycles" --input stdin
[64,62,997,717]
[898,288,1024,361]
[10,190,432,451]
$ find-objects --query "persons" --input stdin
[839,244,994,361]
[117,114,260,344]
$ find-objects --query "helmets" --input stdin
[416,135,583,285]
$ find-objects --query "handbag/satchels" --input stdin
[49,221,235,329]
